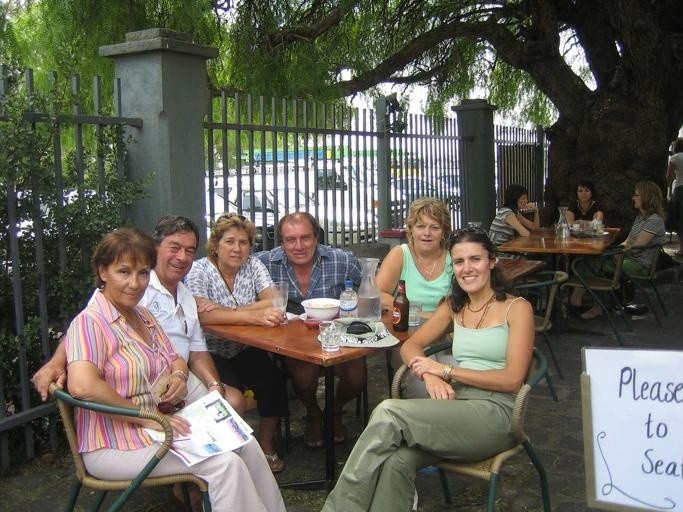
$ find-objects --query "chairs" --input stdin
[389,343,550,511]
[47,383,209,512]
[488,224,675,402]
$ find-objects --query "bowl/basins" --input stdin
[301,298,340,321]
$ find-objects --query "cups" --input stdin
[584,222,594,238]
[408,301,422,327]
[319,321,341,352]
[596,224,605,234]
[573,224,580,233]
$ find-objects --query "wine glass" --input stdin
[270,280,289,326]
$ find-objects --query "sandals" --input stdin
[304,406,322,446]
[333,407,346,442]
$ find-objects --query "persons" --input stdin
[318,227,536,512]
[564,181,604,272]
[182,214,290,472]
[250,211,364,448]
[665,137,683,256]
[487,183,539,260]
[63,227,288,512]
[30,214,247,512]
[561,181,666,319]
[373,196,460,398]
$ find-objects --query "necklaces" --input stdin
[413,255,437,277]
[460,293,497,329]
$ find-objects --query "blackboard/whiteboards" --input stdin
[581,346,683,512]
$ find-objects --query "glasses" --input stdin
[632,195,641,196]
[217,216,246,223]
[157,384,185,414]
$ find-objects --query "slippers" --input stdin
[581,308,602,319]
[563,296,582,307]
[265,452,284,472]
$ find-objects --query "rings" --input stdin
[268,315,272,320]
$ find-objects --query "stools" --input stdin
[277,358,368,448]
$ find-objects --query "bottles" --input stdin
[356,257,382,321]
[339,280,359,317]
[392,280,409,331]
[556,207,571,241]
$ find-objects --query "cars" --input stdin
[384,174,462,211]
[204,181,378,233]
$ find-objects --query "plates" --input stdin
[303,319,322,327]
[299,313,339,321]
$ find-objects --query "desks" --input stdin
[201,311,453,492]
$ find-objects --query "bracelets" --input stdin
[206,382,223,388]
[442,364,452,379]
[171,370,187,379]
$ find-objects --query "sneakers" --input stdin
[169,484,204,511]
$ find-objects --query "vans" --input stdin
[268,161,407,228]
